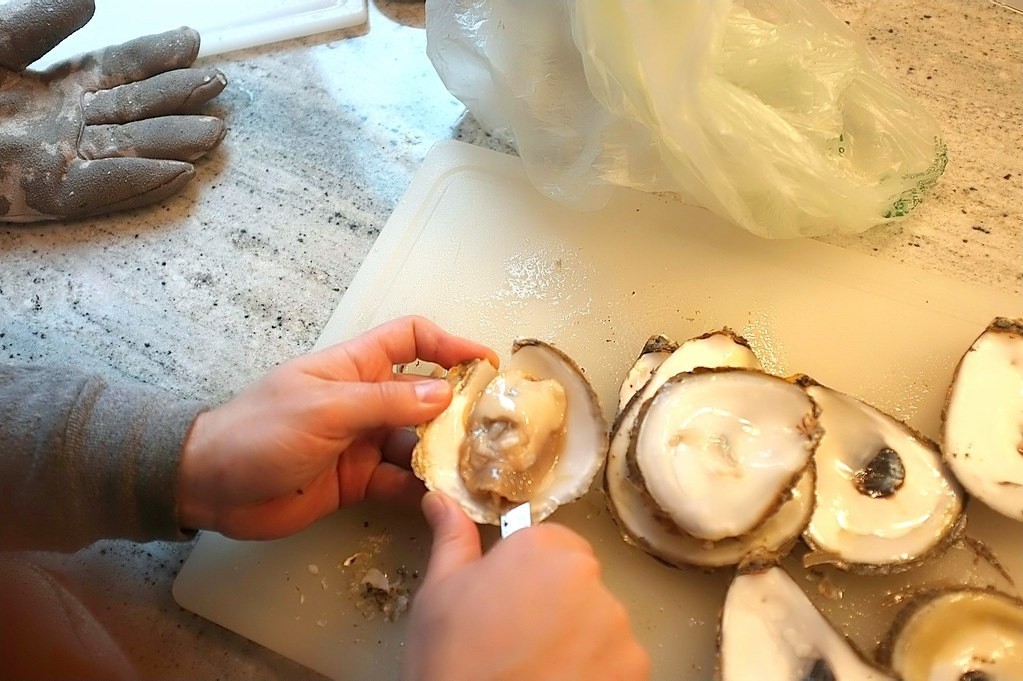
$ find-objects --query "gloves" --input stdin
[0,0,227,223]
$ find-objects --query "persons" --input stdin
[0,315,650,681]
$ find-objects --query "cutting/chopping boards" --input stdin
[0,0,368,80]
[174,140,1023,681]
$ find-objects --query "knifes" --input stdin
[498,495,531,539]
